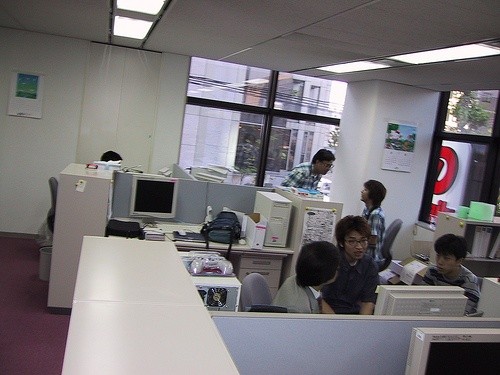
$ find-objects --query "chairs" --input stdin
[376,219,403,273]
[38,177,58,254]
[240,272,273,312]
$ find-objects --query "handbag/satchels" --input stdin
[203,211,241,243]
[106,219,144,240]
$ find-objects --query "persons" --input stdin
[101,151,122,162]
[423,234,480,316]
[281,149,335,189]
[321,215,380,315]
[360,180,387,268]
[274,241,342,314]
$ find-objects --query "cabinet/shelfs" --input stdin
[236,257,283,299]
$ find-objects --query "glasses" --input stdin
[321,162,334,170]
[345,238,370,246]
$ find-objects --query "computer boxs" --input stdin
[253,191,292,248]
[193,275,242,313]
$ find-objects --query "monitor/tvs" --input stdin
[405,328,500,375]
[130,175,178,222]
[374,286,468,317]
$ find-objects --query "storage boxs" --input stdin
[456,201,496,221]
